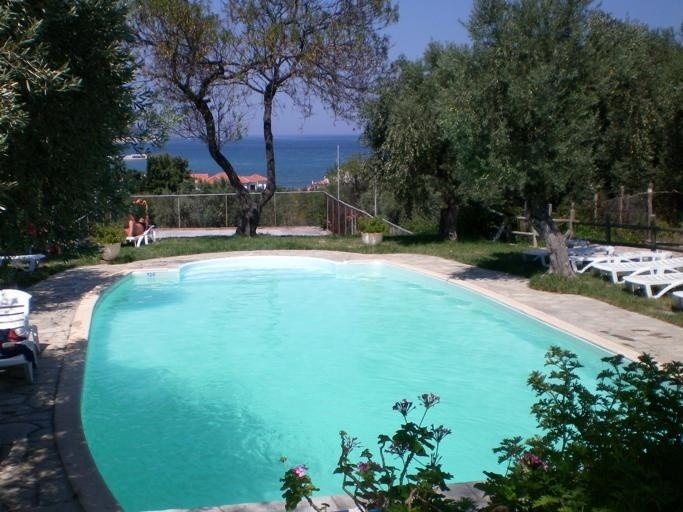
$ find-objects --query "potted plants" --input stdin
[358,213,390,245]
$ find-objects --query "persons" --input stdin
[123,198,150,239]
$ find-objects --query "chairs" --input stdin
[126,223,156,247]
[0,288,41,384]
[0,254,46,272]
[524,242,683,310]
